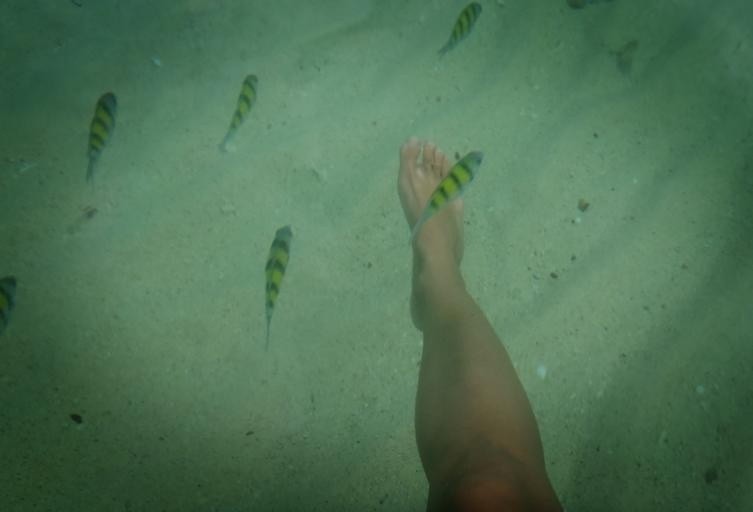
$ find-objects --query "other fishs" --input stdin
[84,90,119,184]
[264,224,294,342]
[0,277,18,336]
[436,2,482,59]
[220,73,259,151]
[409,148,483,245]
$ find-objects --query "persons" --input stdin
[396,136,570,512]
